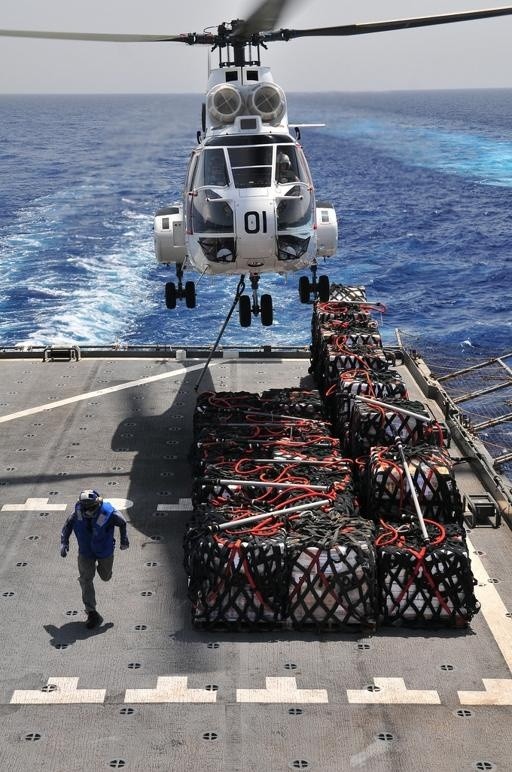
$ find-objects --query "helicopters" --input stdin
[0,0,512,328]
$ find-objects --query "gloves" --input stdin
[119,536,130,551]
[59,542,70,558]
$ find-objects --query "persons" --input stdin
[200,148,300,186]
[57,489,129,630]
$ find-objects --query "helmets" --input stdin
[77,489,102,520]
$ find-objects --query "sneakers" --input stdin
[83,610,102,629]
